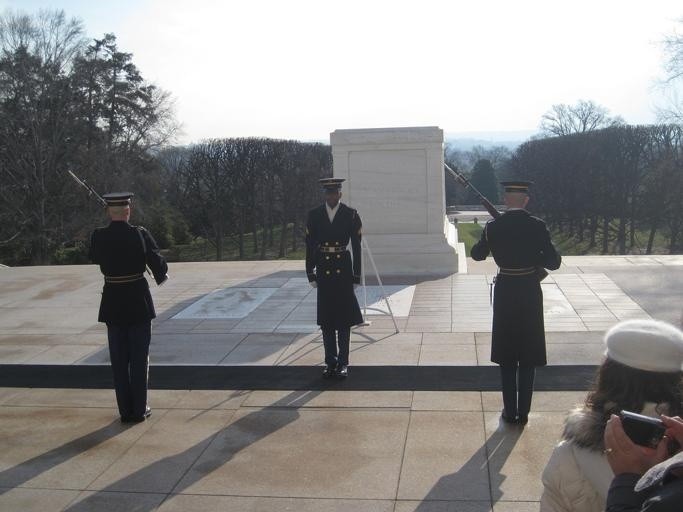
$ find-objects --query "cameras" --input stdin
[620,410,667,449]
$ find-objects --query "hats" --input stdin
[102,191,133,205]
[497,180,533,192]
[318,178,346,187]
[602,318,682,375]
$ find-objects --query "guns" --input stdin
[444,164,549,282]
[68,170,169,285]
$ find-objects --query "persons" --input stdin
[540,319,683,512]
[305,178,364,378]
[470,181,561,425]
[90,192,168,423]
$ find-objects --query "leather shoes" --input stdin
[120,406,152,422]
[503,407,529,425]
[322,362,348,378]
